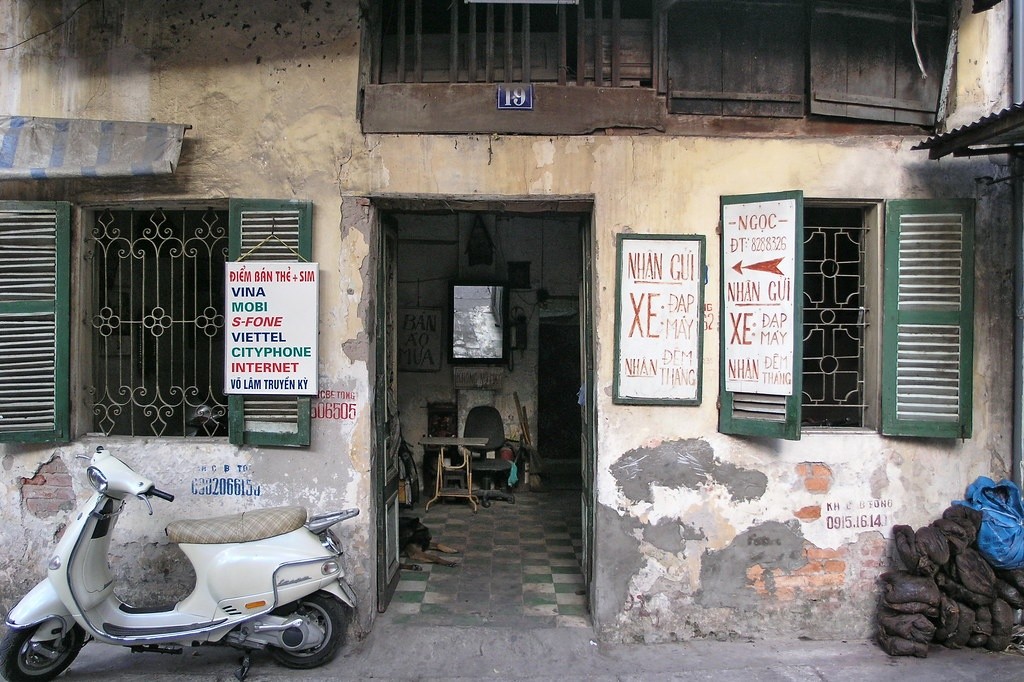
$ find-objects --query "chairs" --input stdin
[463,405,515,507]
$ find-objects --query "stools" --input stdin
[441,469,465,504]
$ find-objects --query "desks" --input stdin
[418,437,491,513]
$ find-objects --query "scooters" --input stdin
[0,444,361,682]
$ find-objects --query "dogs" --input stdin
[399,517,459,572]
